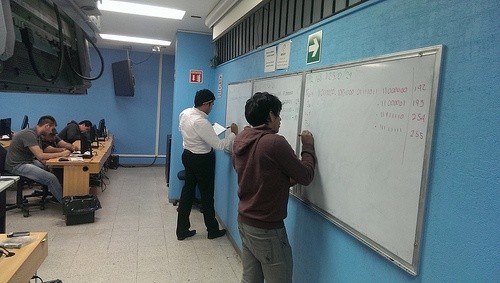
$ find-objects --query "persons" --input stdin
[58,120,92,144]
[232,92,316,283]
[4,116,63,205]
[33,128,75,171]
[176,89,238,241]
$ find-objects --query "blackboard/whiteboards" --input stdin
[224,70,304,198]
[296,42,446,277]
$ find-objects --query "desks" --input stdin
[46,136,113,197]
[0,140,11,150]
[0,231,48,283]
[0,176,20,234]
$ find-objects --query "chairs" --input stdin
[0,145,52,218]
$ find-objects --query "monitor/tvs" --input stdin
[21,115,29,130]
[0,0,91,95]
[0,118,12,136]
[80,119,107,158]
[111,59,135,97]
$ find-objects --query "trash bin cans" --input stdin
[65,194,96,226]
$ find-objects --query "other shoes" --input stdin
[49,197,59,204]
[208,229,226,239]
[177,230,196,240]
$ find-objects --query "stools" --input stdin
[173,170,204,213]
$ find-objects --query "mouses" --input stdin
[59,158,68,161]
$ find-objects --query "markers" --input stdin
[298,133,313,136]
[227,126,231,128]
